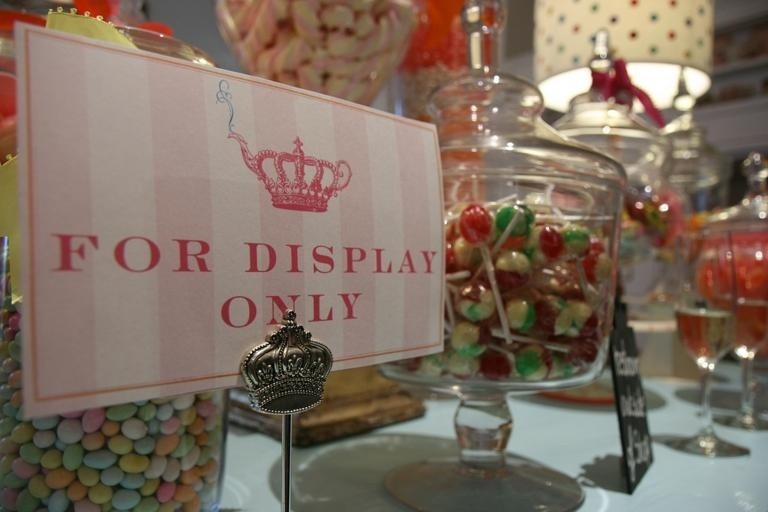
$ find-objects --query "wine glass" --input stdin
[664,227,752,460]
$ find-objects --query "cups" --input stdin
[216,2,416,107]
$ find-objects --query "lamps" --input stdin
[531,0,718,114]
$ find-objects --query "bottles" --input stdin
[544,28,716,319]
[377,70,627,512]
[697,149,766,436]
[2,1,232,512]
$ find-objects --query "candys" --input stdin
[215,0,421,106]
[0,239,225,512]
[390,184,615,382]
